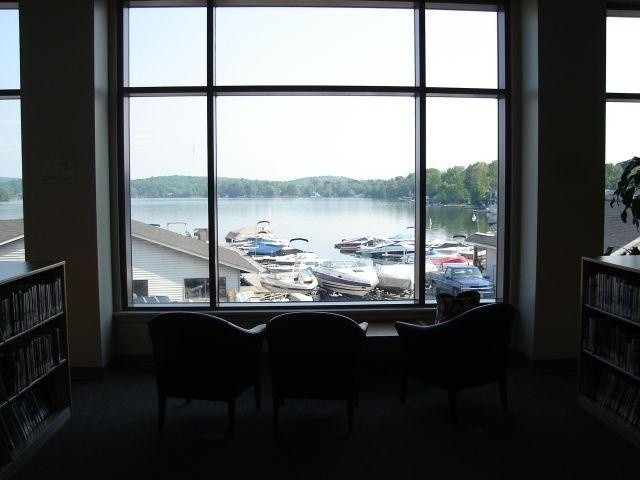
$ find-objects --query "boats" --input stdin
[225,221,486,298]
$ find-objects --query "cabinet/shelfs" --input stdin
[0,261,74,480]
[577,256,640,446]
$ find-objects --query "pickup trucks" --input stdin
[429,264,495,299]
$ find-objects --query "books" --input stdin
[583,271,639,433]
[0,277,65,471]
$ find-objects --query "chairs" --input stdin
[149,311,267,439]
[265,313,369,433]
[394,304,516,413]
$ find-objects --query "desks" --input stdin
[248,319,428,345]
